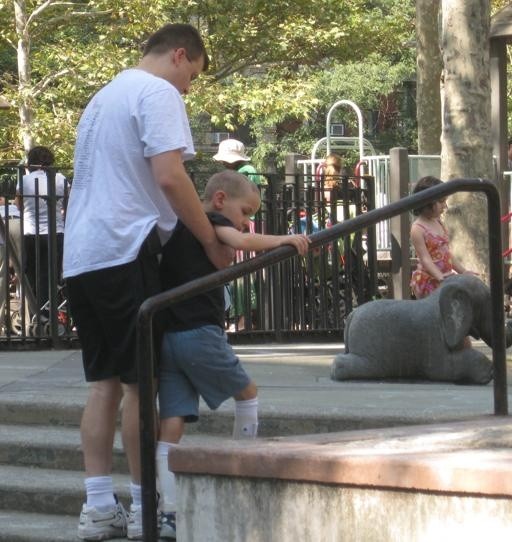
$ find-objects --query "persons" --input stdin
[211,138,263,333]
[286,183,369,331]
[0,196,27,332]
[409,176,480,348]
[156,167,313,504]
[508,135,512,170]
[15,146,70,326]
[322,153,362,206]
[62,23,238,541]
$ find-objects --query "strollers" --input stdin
[28,291,74,340]
[287,210,382,329]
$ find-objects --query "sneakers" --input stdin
[78,503,176,539]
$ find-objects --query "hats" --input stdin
[212,138,252,164]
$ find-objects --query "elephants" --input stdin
[330,273,512,386]
